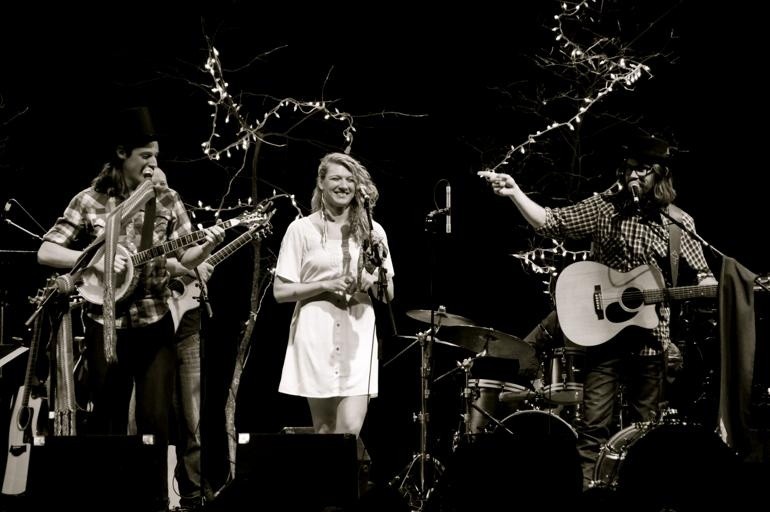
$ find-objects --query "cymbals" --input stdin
[454,324,540,375]
[406,310,476,327]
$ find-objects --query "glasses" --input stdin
[624,165,651,177]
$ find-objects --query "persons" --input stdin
[271,151,396,441]
[475,130,718,494]
[125,167,217,510]
[525,307,587,389]
[35,134,229,510]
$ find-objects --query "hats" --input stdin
[117,106,154,151]
[621,136,673,162]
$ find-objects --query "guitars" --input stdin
[551,260,770,347]
[167,209,277,334]
[0,275,59,495]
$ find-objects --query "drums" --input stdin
[492,412,583,512]
[541,348,586,404]
[588,410,744,511]
[467,356,528,393]
[463,380,535,436]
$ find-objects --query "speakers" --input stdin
[233,431,364,511]
[25,433,169,511]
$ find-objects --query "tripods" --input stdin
[386,330,446,497]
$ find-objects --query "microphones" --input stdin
[143,165,155,181]
[627,179,641,203]
[445,185,452,234]
